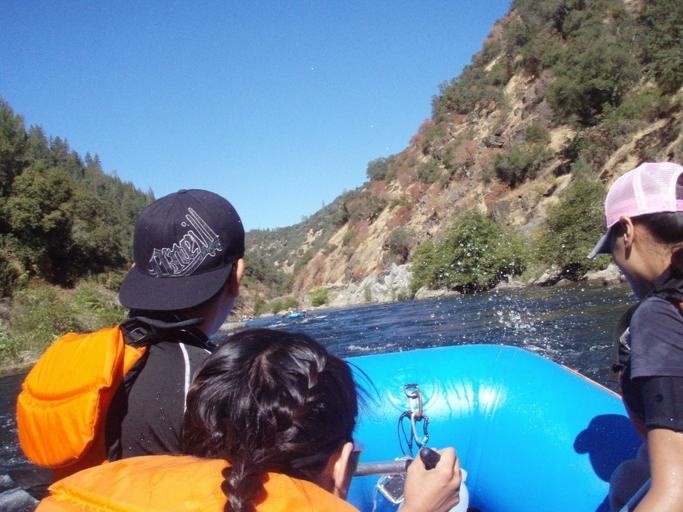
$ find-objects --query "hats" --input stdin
[586,160,682,261]
[119,189,245,311]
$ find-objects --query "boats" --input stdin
[238,341,641,512]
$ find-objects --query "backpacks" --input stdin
[14,325,175,478]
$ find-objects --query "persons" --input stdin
[586,162,683,512]
[31,329,462,512]
[19,189,246,481]
